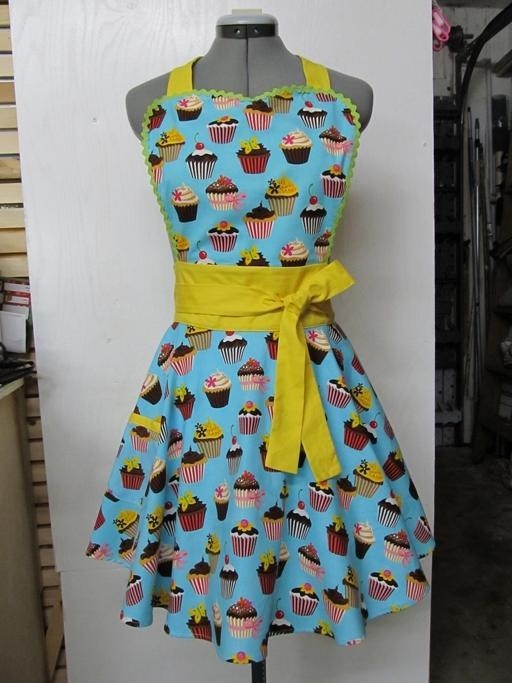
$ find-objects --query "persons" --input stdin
[85,9,436,665]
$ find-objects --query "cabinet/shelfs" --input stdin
[479,103,512,449]
[429,102,476,448]
